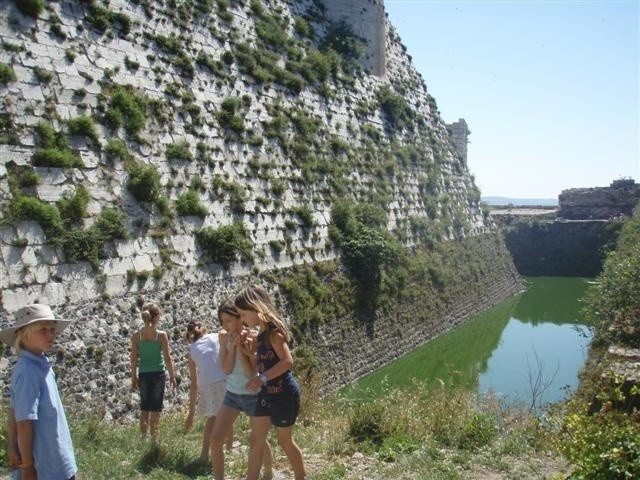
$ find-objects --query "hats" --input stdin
[0,304,72,346]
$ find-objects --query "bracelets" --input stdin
[258,372,270,386]
[16,458,35,468]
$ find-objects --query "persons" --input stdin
[232,285,309,480]
[185,320,234,462]
[210,293,276,480]
[0,303,81,479]
[130,302,178,443]
